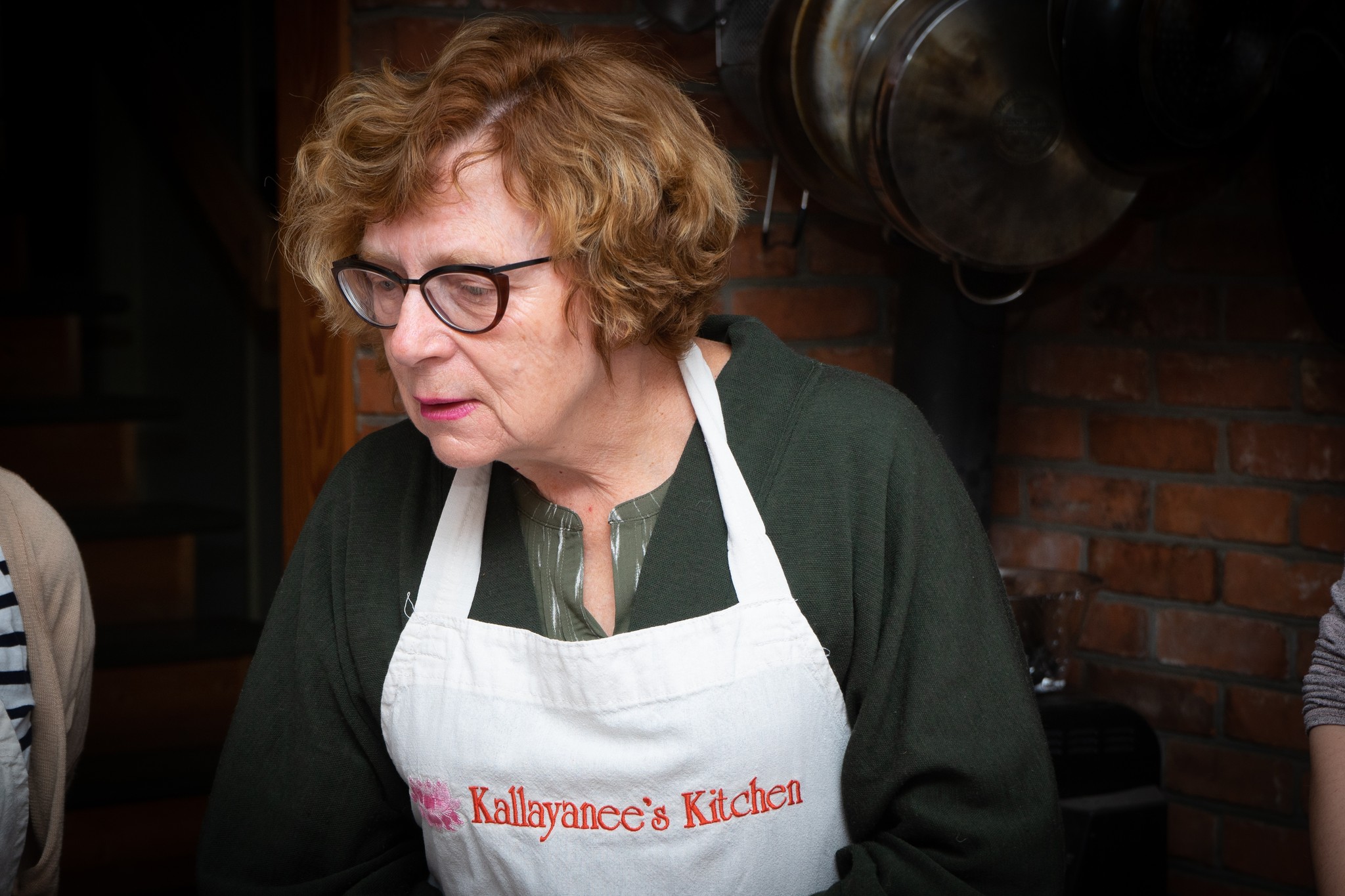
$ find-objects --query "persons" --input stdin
[1302,571,1345,896]
[197,11,1060,896]
[0,467,97,896]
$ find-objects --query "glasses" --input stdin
[331,252,581,331]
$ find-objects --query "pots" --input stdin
[665,0,1165,310]
